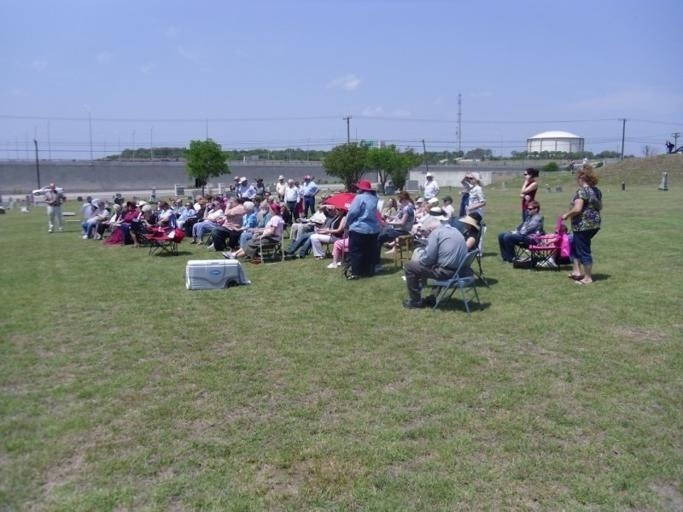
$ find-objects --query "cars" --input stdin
[31,184,64,197]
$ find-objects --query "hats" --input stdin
[136,194,221,214]
[232,175,311,184]
[268,203,281,215]
[350,179,377,193]
[425,171,434,178]
[465,171,483,184]
[424,206,450,221]
[396,191,453,204]
[457,214,482,232]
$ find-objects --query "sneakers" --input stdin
[327,262,342,269]
[402,296,436,309]
[189,237,218,252]
[221,252,238,260]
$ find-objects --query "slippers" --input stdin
[567,269,593,286]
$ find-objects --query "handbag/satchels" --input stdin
[558,232,575,259]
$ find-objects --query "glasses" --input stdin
[525,206,537,212]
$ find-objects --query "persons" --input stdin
[43,183,66,233]
[82,173,320,260]
[282,173,486,309]
[561,166,602,285]
[546,224,573,265]
[520,168,539,222]
[498,201,544,262]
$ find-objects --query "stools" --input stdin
[64,219,81,230]
